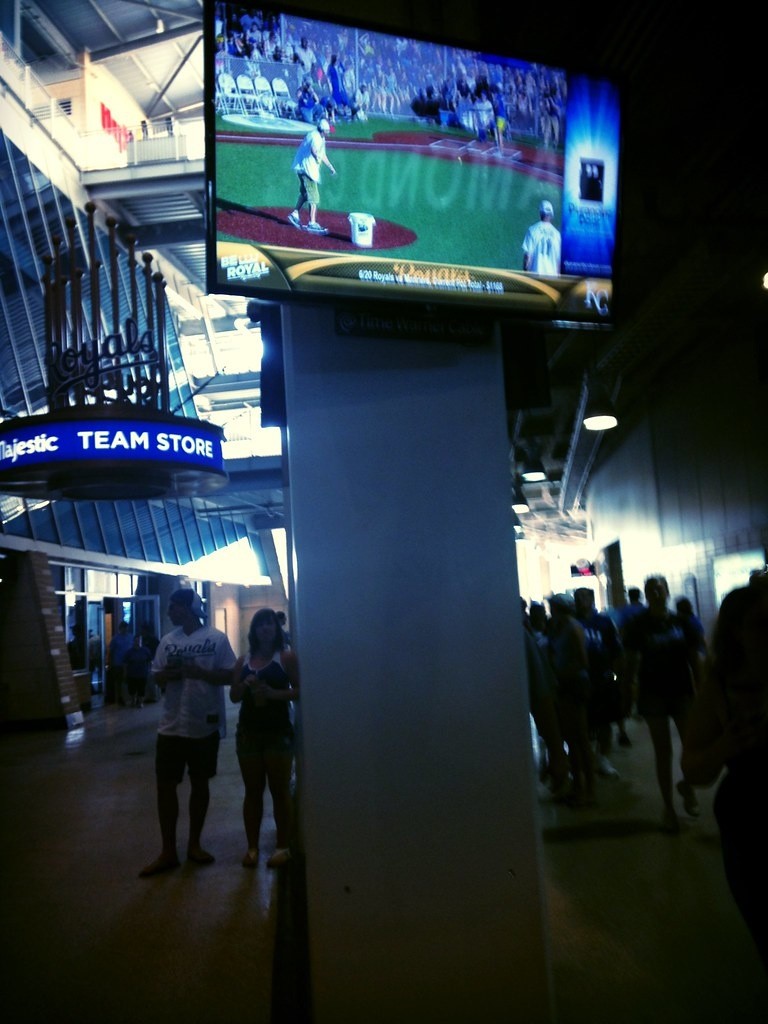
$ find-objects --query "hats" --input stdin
[319,120,330,134]
[170,588,208,619]
[539,200,554,219]
[551,594,575,610]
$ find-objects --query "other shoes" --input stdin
[676,780,701,817]
[138,855,179,878]
[267,848,292,868]
[187,849,215,867]
[287,214,303,233]
[304,222,329,234]
[242,847,258,867]
[664,807,677,827]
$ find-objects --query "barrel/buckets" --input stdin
[348,213,377,248]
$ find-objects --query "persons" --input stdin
[227,608,304,868]
[215,1,570,158]
[285,117,337,232]
[679,586,768,974]
[134,588,241,878]
[517,575,714,834]
[106,619,163,707]
[520,199,563,276]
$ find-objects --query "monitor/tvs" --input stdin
[202,0,627,331]
